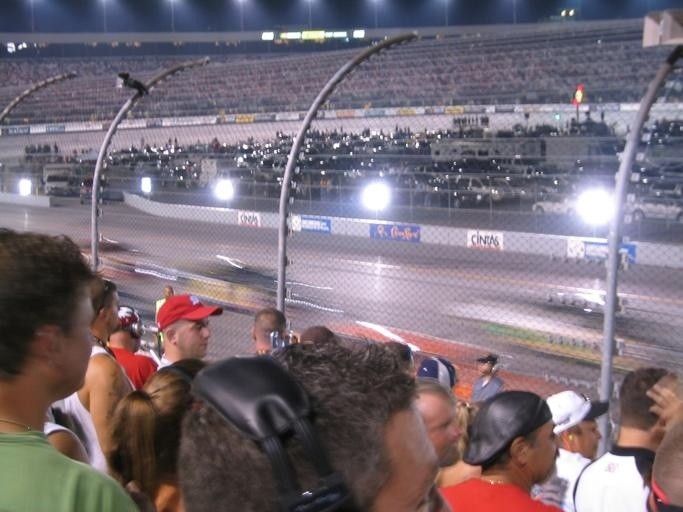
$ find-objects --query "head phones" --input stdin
[119,305,143,338]
[491,355,500,374]
[191,355,357,512]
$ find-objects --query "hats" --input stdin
[545,388,611,437]
[477,353,497,364]
[462,387,554,468]
[155,293,225,333]
[417,354,459,389]
[116,305,141,330]
